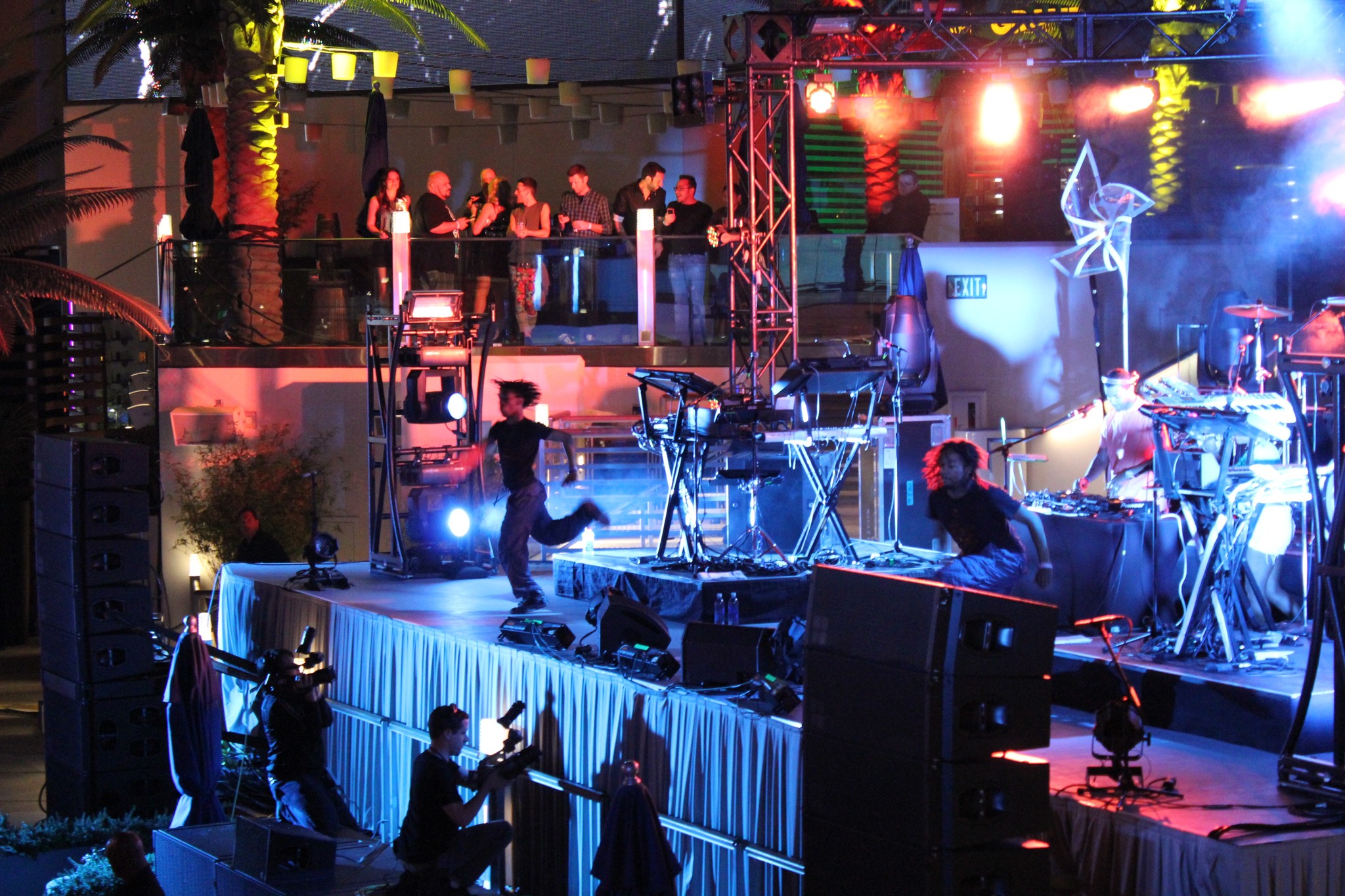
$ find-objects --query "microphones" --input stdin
[1068,398,1102,418]
[1072,613,1124,631]
[880,339,902,351]
[1238,334,1255,346]
[814,338,851,354]
[746,351,760,380]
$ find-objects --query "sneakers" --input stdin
[557,334,594,344]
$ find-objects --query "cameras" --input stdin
[479,743,541,782]
[294,665,339,693]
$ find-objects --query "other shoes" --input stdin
[275,801,282,819]
[522,336,533,346]
[584,498,611,526]
[510,596,549,614]
[470,329,481,341]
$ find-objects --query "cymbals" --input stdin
[1221,303,1294,318]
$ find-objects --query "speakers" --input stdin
[799,562,1061,896]
[597,597,672,659]
[725,450,821,553]
[586,585,628,627]
[32,434,155,817]
[682,621,769,690]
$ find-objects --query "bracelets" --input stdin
[588,221,592,230]
[1039,562,1054,570]
[455,221,460,230]
[469,215,476,222]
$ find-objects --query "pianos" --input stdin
[1151,391,1296,426]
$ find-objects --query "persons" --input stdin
[257,649,372,838]
[875,170,932,240]
[613,161,668,262]
[103,832,167,896]
[370,168,413,316]
[662,174,717,348]
[416,171,470,289]
[480,374,612,615]
[393,706,514,889]
[462,167,499,231]
[1072,367,1172,513]
[1224,435,1303,630]
[553,164,614,326]
[708,182,767,341]
[470,175,514,342]
[918,439,1054,594]
[504,176,551,337]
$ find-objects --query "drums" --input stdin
[1305,406,1327,442]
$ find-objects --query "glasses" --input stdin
[673,187,690,191]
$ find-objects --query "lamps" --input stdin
[295,625,316,655]
[496,701,526,728]
